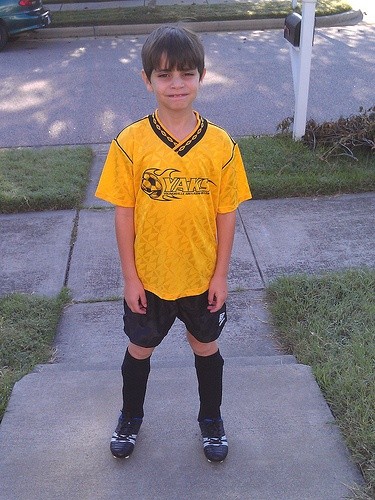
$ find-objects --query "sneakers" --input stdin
[110,410,143,459]
[198,412,228,464]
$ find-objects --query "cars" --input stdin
[0,0,52,52]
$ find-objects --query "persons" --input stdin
[95,26,253,461]
